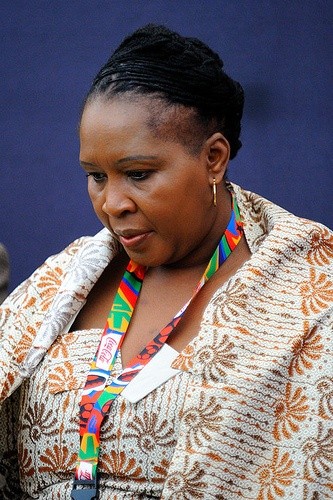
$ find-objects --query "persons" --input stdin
[0,23,333,499]
